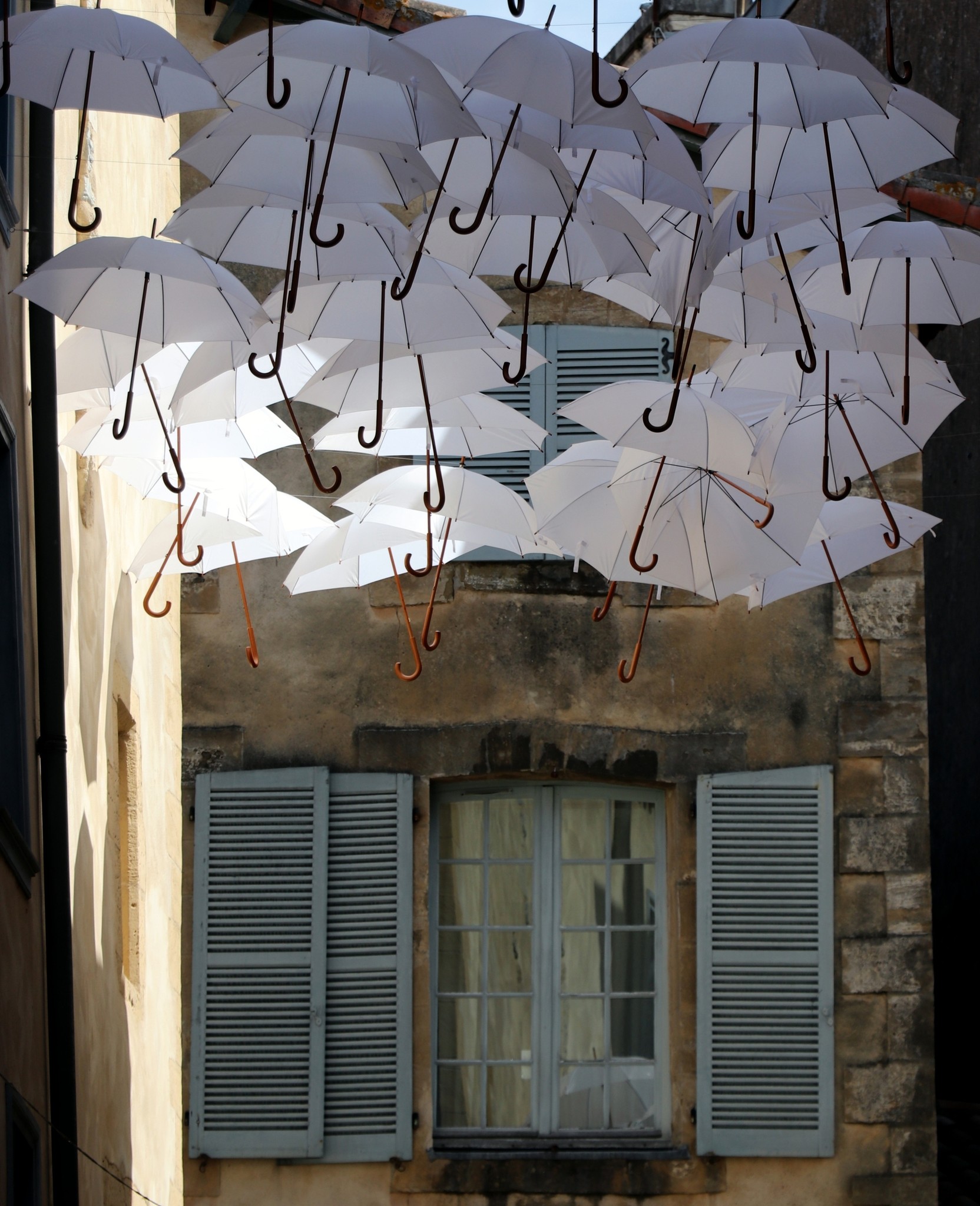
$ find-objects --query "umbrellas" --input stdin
[0,0,234,233]
[7,213,275,438]
[66,2,980,681]
